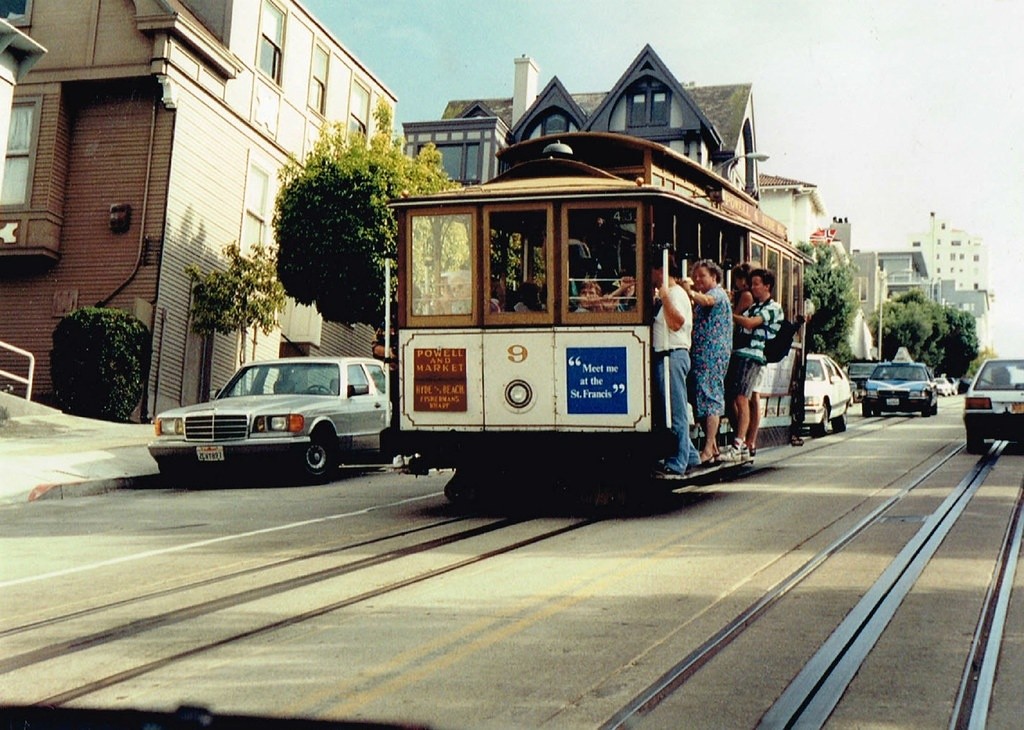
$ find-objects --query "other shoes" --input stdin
[650,465,682,480]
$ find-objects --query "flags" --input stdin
[810,229,837,244]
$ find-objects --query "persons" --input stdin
[415,238,784,477]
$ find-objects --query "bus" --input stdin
[380,132,814,502]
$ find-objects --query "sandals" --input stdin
[696,456,714,467]
[713,453,722,465]
[750,446,756,456]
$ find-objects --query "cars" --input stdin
[935,377,973,397]
[802,353,852,436]
[962,359,1024,452]
[148,357,394,485]
[862,362,938,417]
[847,363,878,402]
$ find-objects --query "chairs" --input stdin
[330,379,339,391]
[273,381,295,395]
[990,367,1011,385]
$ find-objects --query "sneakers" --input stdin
[716,445,741,462]
[719,444,750,460]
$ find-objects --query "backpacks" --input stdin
[762,300,794,362]
